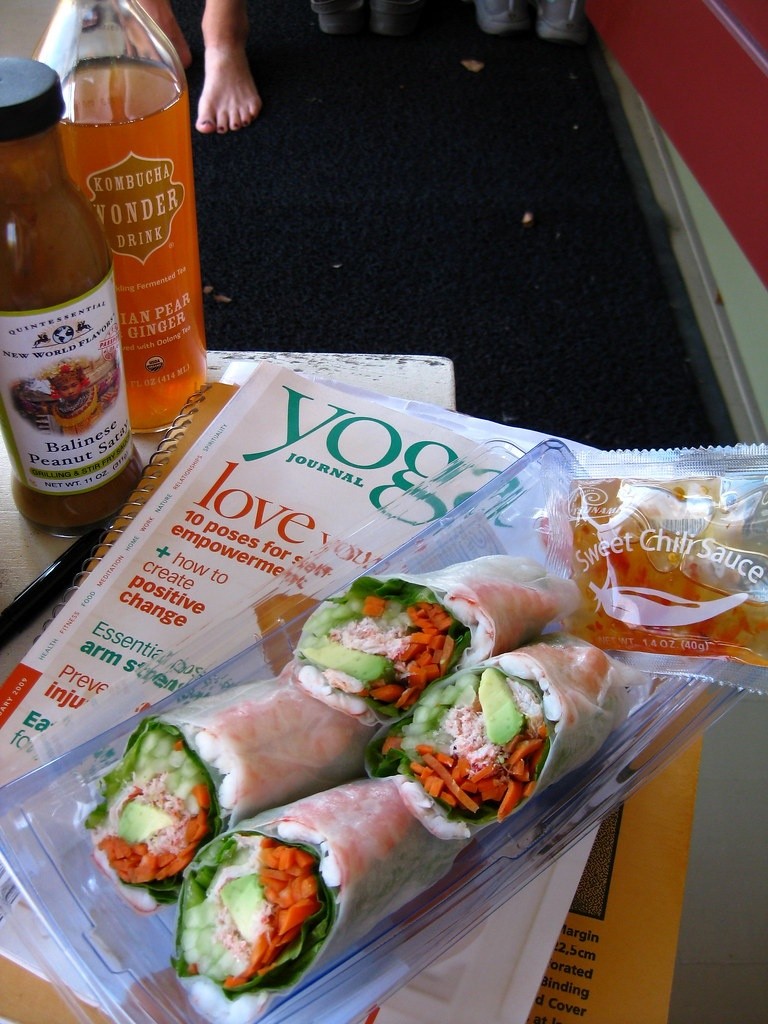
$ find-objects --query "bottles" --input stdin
[0,55,143,538]
[33,1,207,433]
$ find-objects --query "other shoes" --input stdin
[536,0,588,44]
[310,0,365,33]
[475,0,531,34]
[369,0,422,37]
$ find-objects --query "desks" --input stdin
[0,348,459,1024]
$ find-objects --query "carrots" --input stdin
[97,584,609,987]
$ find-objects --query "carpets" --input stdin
[165,0,743,454]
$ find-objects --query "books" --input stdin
[0,365,701,1024]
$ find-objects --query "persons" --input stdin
[124,0,262,133]
